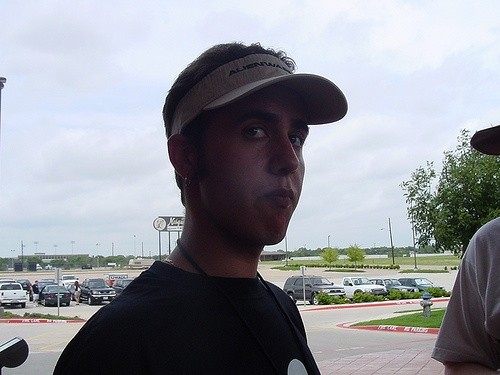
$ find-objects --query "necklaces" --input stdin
[177,239,207,275]
[52,42,348,375]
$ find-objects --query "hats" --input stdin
[470,124,500,157]
[167,50,348,145]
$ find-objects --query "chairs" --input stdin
[354,280,358,284]
[345,281,349,286]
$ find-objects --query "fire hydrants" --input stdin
[420,291,435,317]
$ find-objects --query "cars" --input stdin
[0,283,30,308]
[370,279,418,297]
[75,278,117,304]
[399,279,439,294]
[39,284,72,307]
[1,273,130,300]
[112,278,137,299]
[283,276,345,305]
[338,276,388,300]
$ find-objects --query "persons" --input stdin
[73,281,81,305]
[31,280,40,307]
[431,125,500,375]
